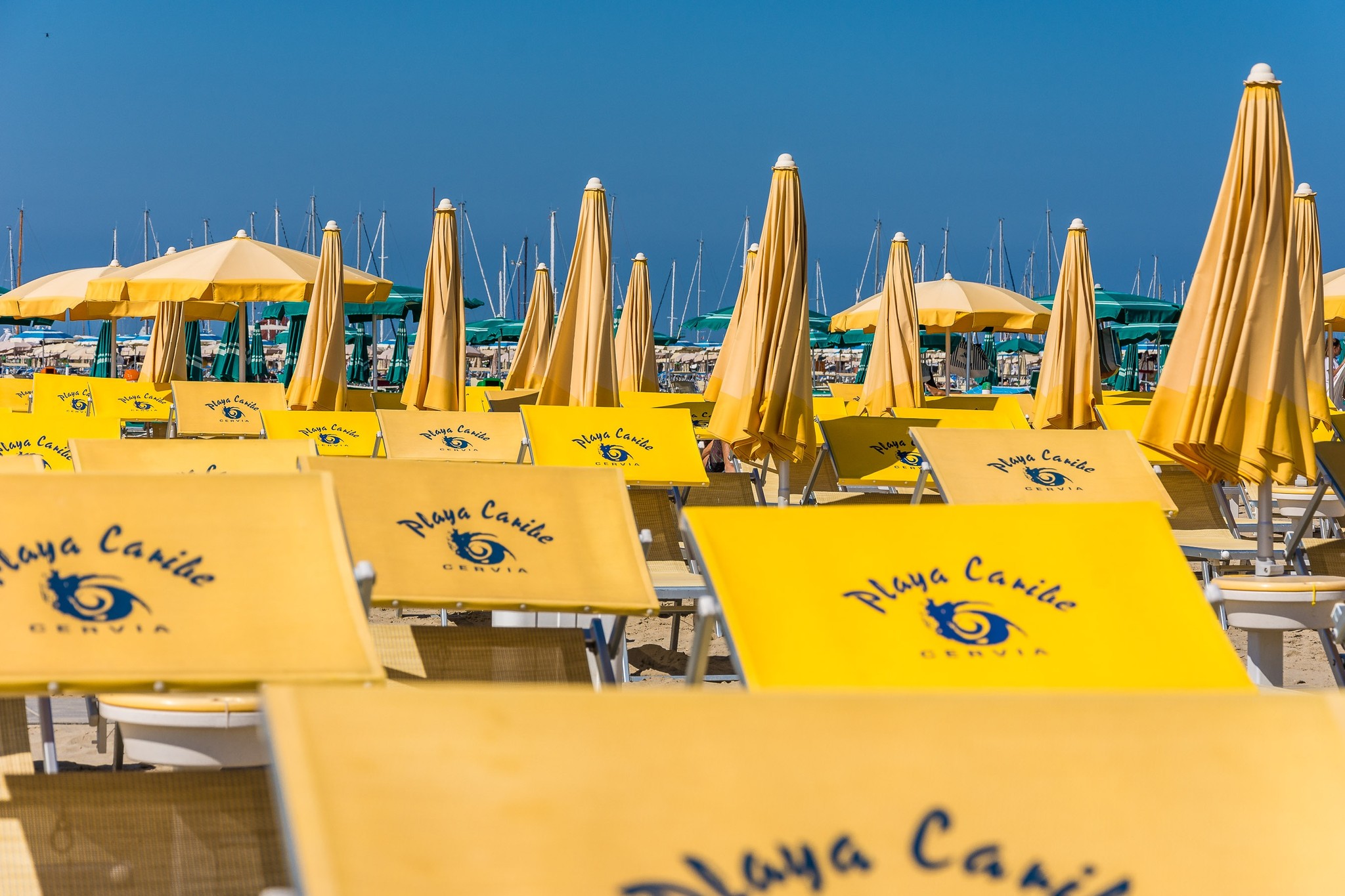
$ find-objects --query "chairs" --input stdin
[1,452,1345,895]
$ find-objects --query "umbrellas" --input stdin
[462,253,681,393]
[707,153,818,504]
[398,198,464,413]
[0,231,471,389]
[1285,182,1345,431]
[285,220,348,412]
[682,231,1182,405]
[1026,219,1106,429]
[532,176,623,406]
[1136,59,1320,487]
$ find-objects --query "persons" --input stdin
[1324,337,1345,397]
[136,356,140,362]
[128,355,135,367]
[270,371,277,379]
[138,351,140,356]
